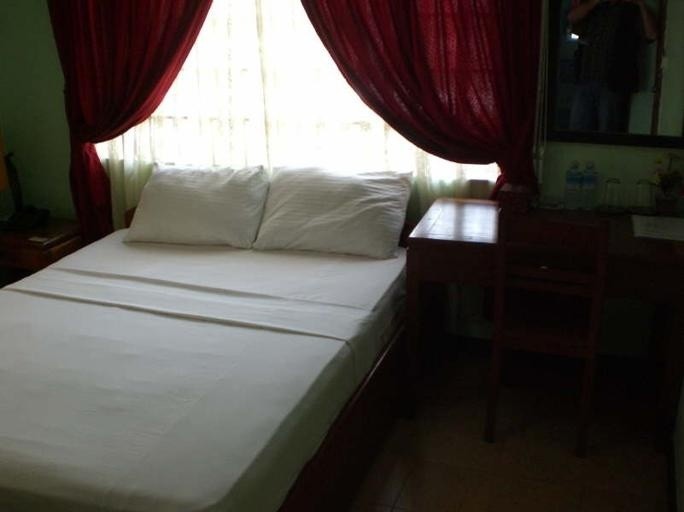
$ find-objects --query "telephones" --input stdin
[7,203,49,230]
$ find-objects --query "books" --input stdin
[23,230,66,248]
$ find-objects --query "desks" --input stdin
[410,195,683,457]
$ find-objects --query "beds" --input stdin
[2,226,405,510]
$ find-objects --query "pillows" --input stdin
[120,166,411,259]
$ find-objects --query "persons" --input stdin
[564,0,659,136]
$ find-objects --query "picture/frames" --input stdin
[526,128,684,220]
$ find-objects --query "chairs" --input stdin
[482,197,614,456]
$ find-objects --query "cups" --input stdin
[600,178,624,212]
[630,179,657,214]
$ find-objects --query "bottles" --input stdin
[582,162,598,209]
[564,160,582,210]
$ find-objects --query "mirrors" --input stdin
[544,0,668,136]
